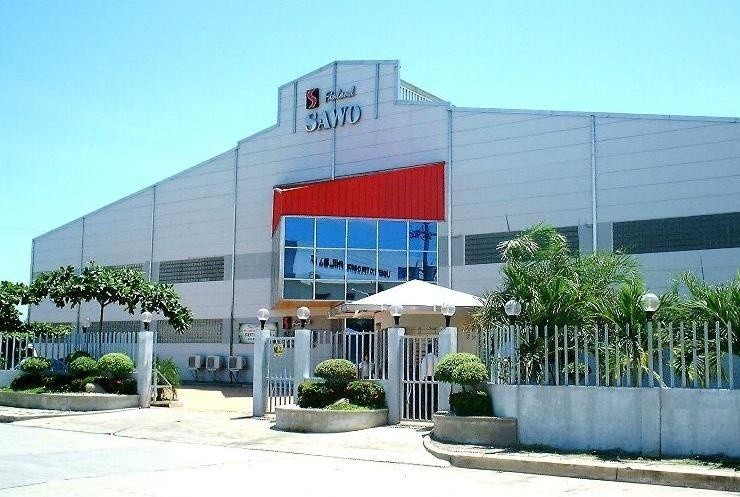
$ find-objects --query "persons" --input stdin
[421,343,438,419]
[359,353,376,380]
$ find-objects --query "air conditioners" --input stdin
[188,353,247,370]
